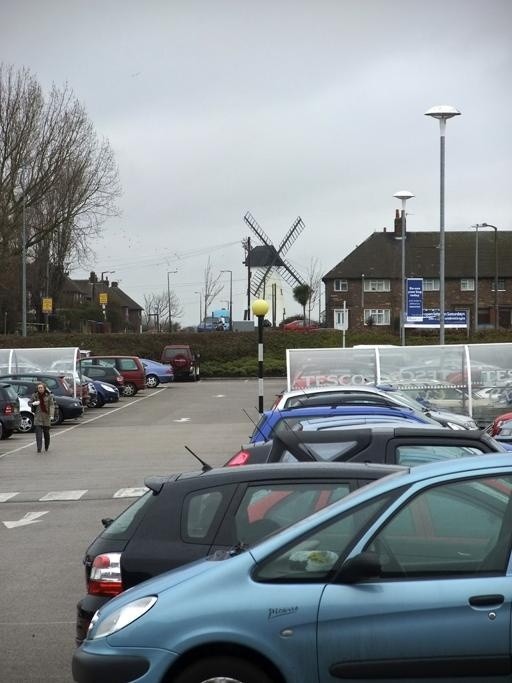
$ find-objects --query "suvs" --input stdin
[160,343,203,382]
[75,461,510,650]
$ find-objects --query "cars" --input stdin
[72,452,512,682]
[282,319,322,330]
[196,313,231,333]
[0,345,178,442]
[219,385,512,545]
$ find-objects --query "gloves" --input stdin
[50,417,54,420]
[33,401,40,406]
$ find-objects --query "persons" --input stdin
[27,382,55,453]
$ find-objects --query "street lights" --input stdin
[391,189,417,347]
[219,299,229,309]
[250,297,271,415]
[22,255,36,336]
[193,291,202,324]
[481,221,500,329]
[220,269,232,331]
[204,294,216,331]
[101,270,116,320]
[422,102,462,344]
[168,270,178,332]
